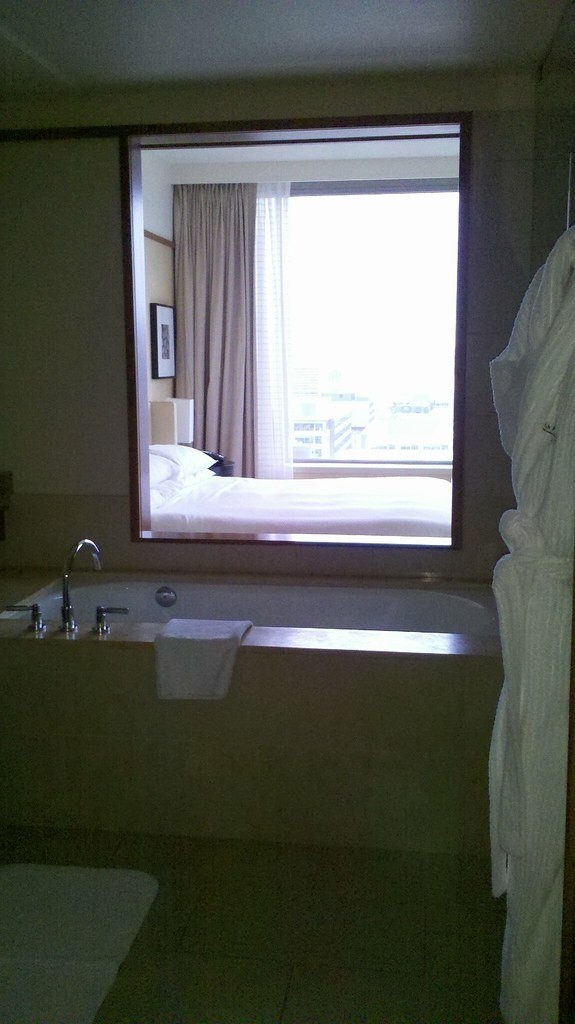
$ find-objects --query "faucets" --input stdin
[61,537,104,627]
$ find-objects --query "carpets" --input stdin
[0,863,161,1024]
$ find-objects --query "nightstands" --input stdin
[209,460,236,477]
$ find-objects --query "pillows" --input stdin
[149,443,219,479]
[175,467,219,488]
[150,479,184,510]
[149,452,178,487]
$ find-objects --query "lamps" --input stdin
[169,398,195,447]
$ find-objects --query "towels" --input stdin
[155,618,255,701]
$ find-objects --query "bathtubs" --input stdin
[0,565,504,858]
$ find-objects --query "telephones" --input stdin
[203,450,225,464]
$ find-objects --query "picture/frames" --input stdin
[149,301,178,379]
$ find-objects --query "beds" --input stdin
[149,477,452,536]
